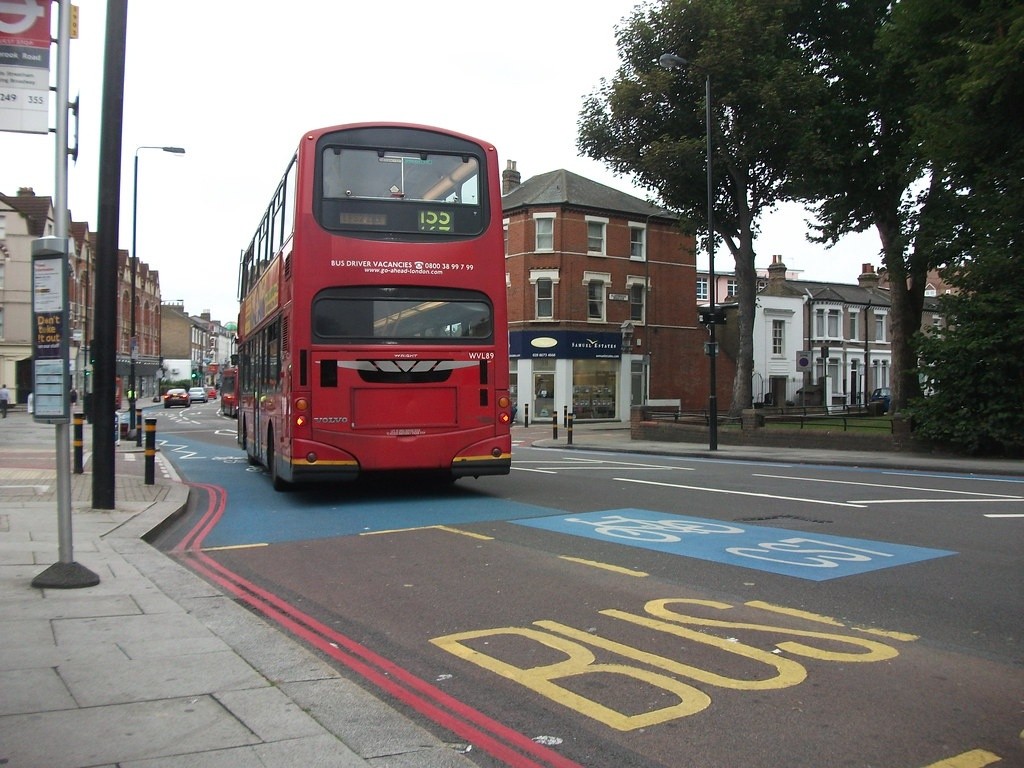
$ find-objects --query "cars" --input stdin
[204,387,217,399]
[164,388,191,408]
[868,387,890,412]
[188,387,208,403]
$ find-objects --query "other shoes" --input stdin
[3,415,6,418]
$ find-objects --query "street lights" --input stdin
[129,147,186,439]
[159,300,185,403]
[659,52,719,449]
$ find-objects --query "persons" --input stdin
[216,382,220,390]
[204,383,207,387]
[127,384,136,408]
[0,385,11,418]
[71,390,77,402]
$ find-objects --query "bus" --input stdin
[230,121,512,492]
[216,366,242,419]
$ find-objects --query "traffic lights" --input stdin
[90,339,95,365]
[192,369,197,381]
